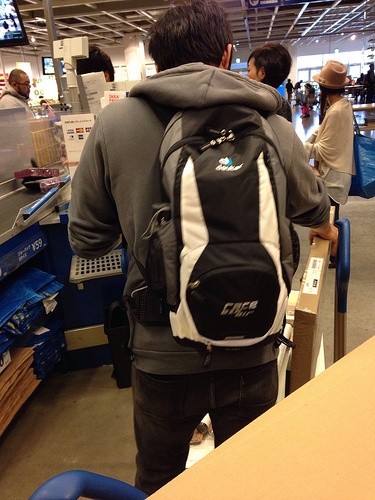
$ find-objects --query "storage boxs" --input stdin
[290,205,337,395]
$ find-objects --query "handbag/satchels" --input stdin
[348,110,375,198]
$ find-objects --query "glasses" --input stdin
[15,82,31,88]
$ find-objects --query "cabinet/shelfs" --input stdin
[353,103,375,133]
[0,108,138,449]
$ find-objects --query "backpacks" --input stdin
[132,105,301,368]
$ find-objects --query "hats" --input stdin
[311,60,351,89]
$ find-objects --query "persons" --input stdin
[302,83,315,117]
[40,100,52,110]
[345,64,375,104]
[246,43,292,123]
[304,61,354,268]
[0,69,31,109]
[294,80,303,91]
[286,79,293,104]
[3,13,17,31]
[68,0,338,497]
[76,46,114,82]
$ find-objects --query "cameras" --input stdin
[0,0,30,49]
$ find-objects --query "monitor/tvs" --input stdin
[42,56,68,75]
